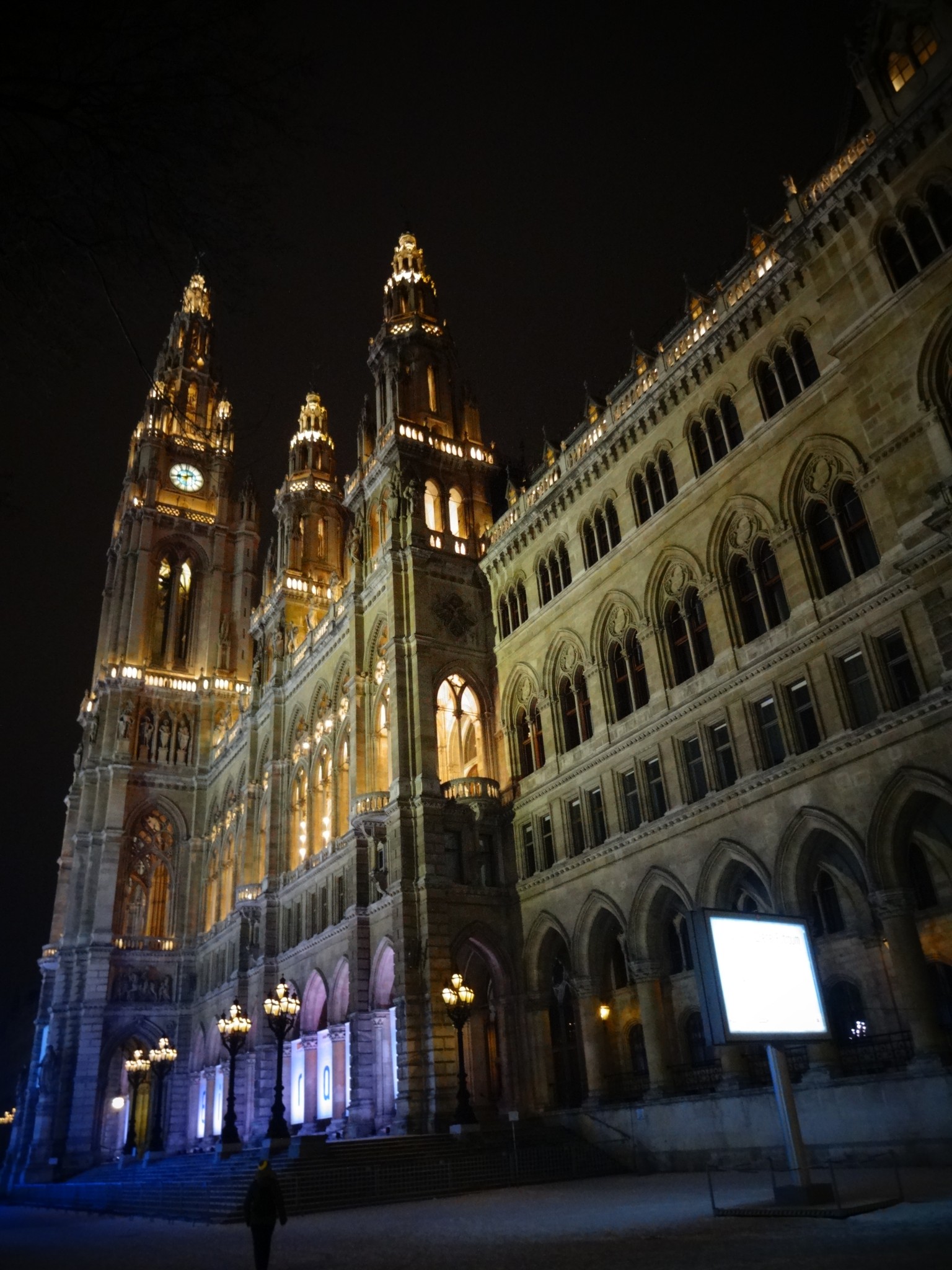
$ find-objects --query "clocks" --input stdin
[169,463,204,493]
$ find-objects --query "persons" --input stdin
[244,1158,288,1270]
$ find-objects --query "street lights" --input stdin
[217,997,254,1157]
[440,972,484,1134]
[118,1048,151,1167]
[260,973,302,1152]
[142,1037,179,1165]
